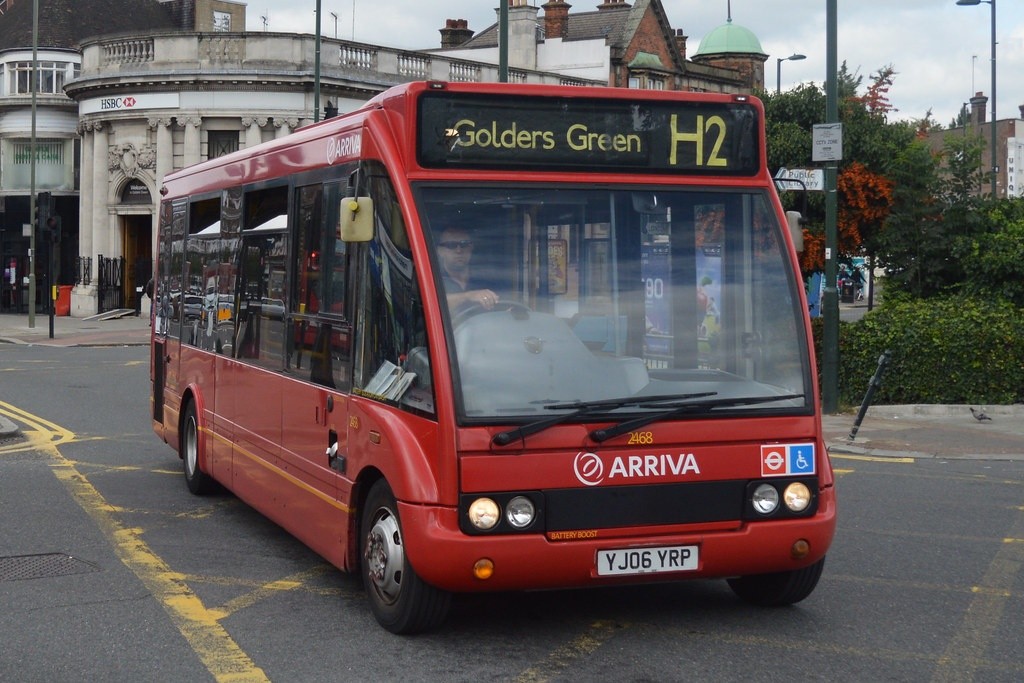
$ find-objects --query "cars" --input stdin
[168,288,179,302]
[260,297,285,321]
[158,294,205,318]
[202,292,234,321]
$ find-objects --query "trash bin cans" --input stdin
[53,285,72,317]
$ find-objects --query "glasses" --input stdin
[440,240,473,249]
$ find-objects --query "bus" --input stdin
[294,250,352,356]
[151,80,836,635]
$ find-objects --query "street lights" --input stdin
[776,53,806,96]
[956,0,998,204]
[972,55,977,97]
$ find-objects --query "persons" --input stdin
[146,279,154,326]
[411,223,501,346]
[839,264,868,301]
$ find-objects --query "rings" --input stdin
[483,296,487,301]
